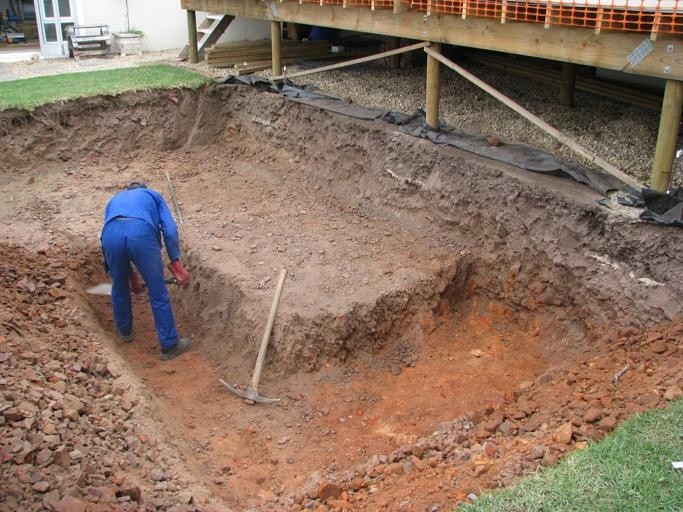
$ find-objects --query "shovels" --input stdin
[85,277,177,295]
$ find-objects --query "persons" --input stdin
[100,181,193,362]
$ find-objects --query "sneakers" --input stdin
[161,338,189,360]
[116,326,131,343]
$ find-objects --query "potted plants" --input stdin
[112,0,146,57]
